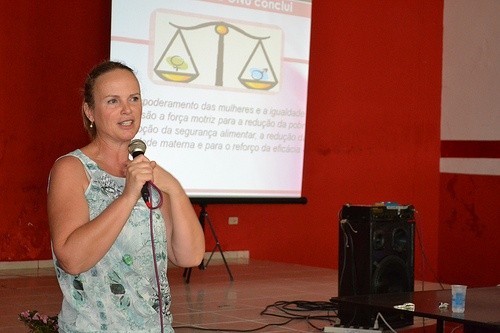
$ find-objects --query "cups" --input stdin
[451,285,467,314]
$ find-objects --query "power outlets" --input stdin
[228,217,238,224]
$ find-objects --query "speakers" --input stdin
[337,205,416,329]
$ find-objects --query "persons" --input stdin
[46,62,206,333]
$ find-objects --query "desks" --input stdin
[329,286,500,333]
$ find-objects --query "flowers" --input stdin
[19,309,58,333]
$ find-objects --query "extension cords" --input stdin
[324,324,383,333]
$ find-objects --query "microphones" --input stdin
[127,139,150,203]
